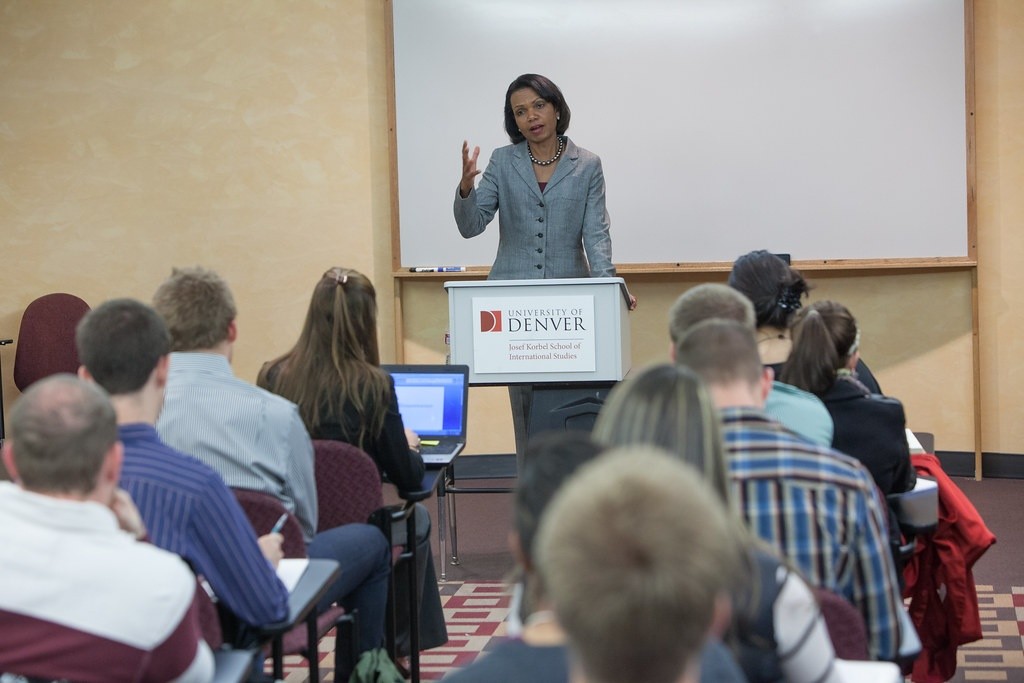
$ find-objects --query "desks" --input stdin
[408,465,446,501]
[885,431,940,533]
[200,556,344,636]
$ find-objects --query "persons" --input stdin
[672,249,925,591]
[439,434,745,683]
[534,444,740,683]
[673,321,903,666]
[258,268,448,675]
[151,265,413,683]
[76,297,290,631]
[594,364,845,683]
[454,75,637,312]
[0,376,220,683]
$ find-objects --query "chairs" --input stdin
[0,290,451,683]
[805,586,870,661]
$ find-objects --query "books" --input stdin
[201,558,310,602]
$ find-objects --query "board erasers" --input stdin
[772,254,790,266]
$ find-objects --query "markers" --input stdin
[408,266,467,272]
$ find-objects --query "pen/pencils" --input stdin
[268,513,288,533]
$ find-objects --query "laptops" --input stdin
[380,364,469,466]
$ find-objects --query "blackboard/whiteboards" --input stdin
[385,0,977,273]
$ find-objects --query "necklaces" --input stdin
[757,333,791,347]
[527,137,563,165]
